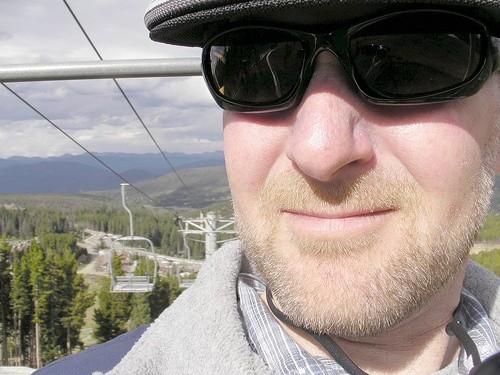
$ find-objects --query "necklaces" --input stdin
[264,282,482,375]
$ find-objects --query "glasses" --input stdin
[199,9,496,113]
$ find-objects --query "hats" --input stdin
[143,0,500,48]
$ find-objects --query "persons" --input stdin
[31,0,500,375]
